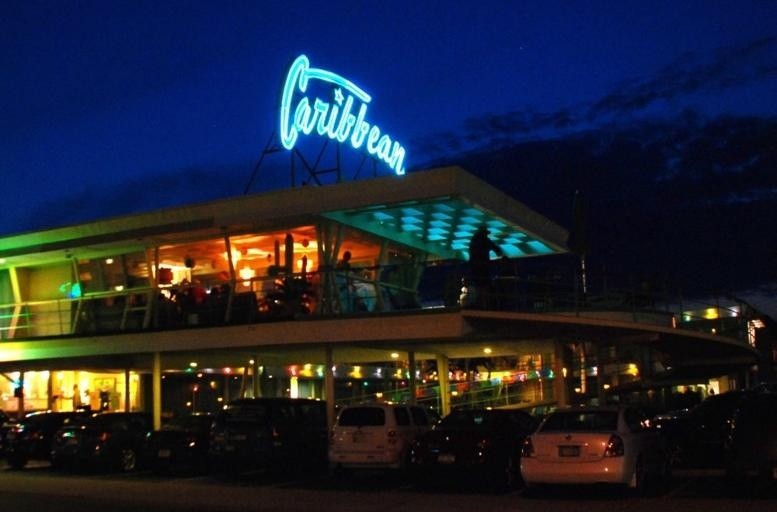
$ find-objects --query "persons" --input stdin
[174,251,354,324]
[466,222,504,263]
